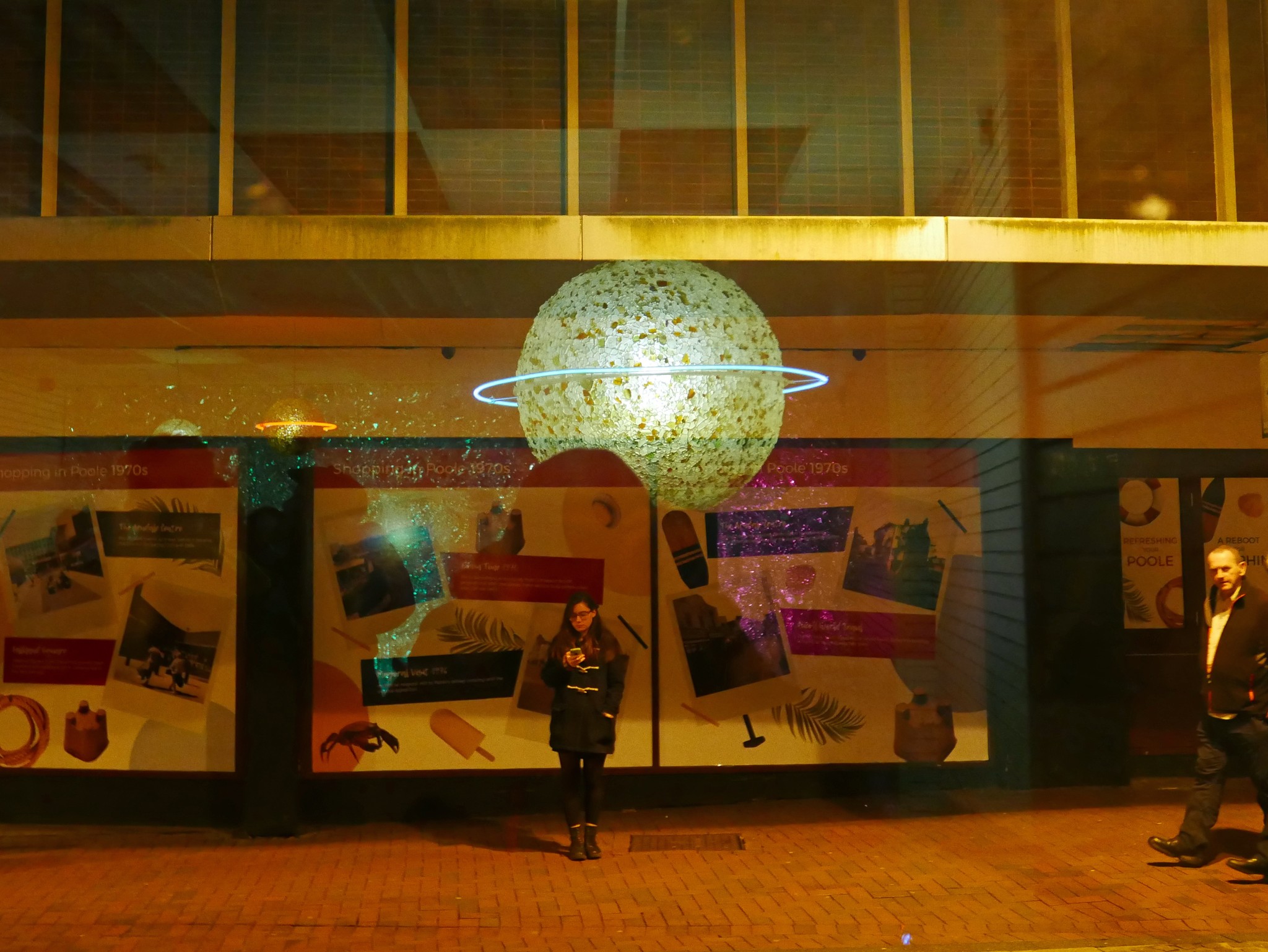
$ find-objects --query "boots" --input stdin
[584,825,602,858]
[569,824,586,860]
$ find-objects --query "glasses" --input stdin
[569,611,591,622]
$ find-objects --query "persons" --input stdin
[543,591,624,861]
[140,644,193,694]
[1145,544,1268,879]
[46,571,73,595]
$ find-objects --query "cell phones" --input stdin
[570,648,582,656]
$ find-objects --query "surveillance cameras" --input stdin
[853,349,866,361]
[441,347,456,359]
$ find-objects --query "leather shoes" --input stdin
[1148,834,1201,866]
[1227,856,1268,877]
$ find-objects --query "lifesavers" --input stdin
[1119,478,1163,527]
